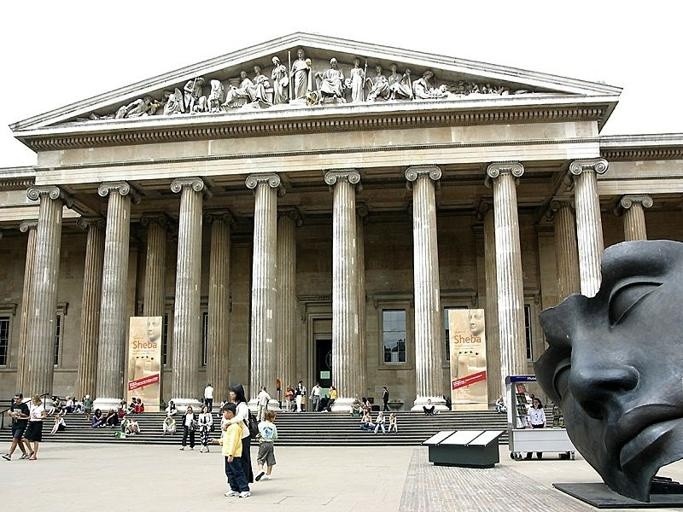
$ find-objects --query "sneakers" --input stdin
[20,451,37,460]
[199,445,209,453]
[179,446,194,450]
[224,489,251,499]
[254,470,273,482]
[1,453,12,461]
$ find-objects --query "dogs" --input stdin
[422,406,435,415]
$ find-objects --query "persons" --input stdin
[422,399,435,416]
[495,382,547,460]
[127,317,161,406]
[532,240,683,502]
[452,309,486,404]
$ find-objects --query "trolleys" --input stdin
[502,373,576,461]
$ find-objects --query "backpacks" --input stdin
[248,408,260,439]
